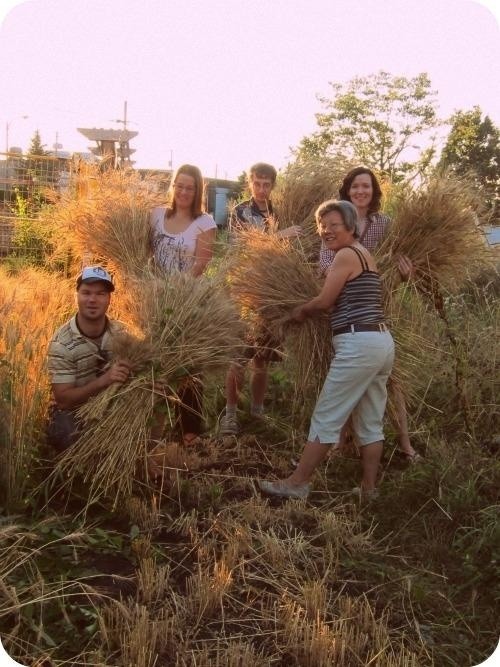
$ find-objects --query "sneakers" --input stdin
[337,487,376,506]
[252,411,274,425]
[261,476,313,500]
[220,418,239,435]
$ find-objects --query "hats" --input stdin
[77,265,116,293]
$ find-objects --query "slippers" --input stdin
[400,449,422,465]
[181,432,203,448]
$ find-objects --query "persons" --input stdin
[221,161,304,445]
[256,197,396,501]
[29,265,175,498]
[317,164,421,462]
[145,162,219,437]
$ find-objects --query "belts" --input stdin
[331,322,390,338]
